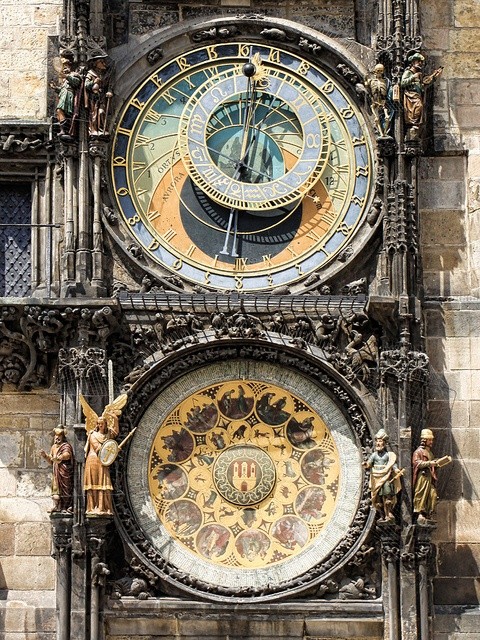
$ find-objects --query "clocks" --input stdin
[91,18,386,300]
[104,330,382,606]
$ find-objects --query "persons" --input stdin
[82,51,112,138]
[360,427,406,527]
[39,423,76,515]
[82,414,123,518]
[111,577,155,601]
[400,53,444,130]
[315,573,381,600]
[410,428,441,525]
[149,380,340,564]
[49,59,83,137]
